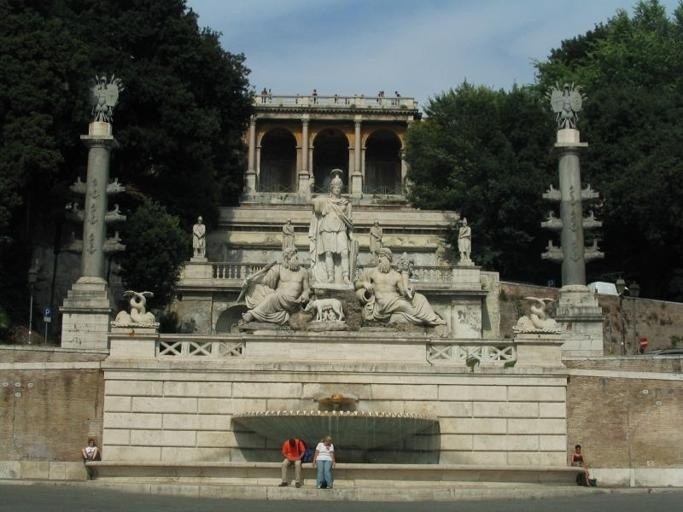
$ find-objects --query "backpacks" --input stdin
[297,439,313,463]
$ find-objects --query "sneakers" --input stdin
[279,482,288,487]
[296,483,300,488]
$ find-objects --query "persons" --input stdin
[313,436,336,489]
[192,216,206,257]
[306,178,353,284]
[282,218,295,250]
[458,217,471,259]
[355,248,447,325]
[571,445,591,487]
[370,220,383,254]
[279,438,306,488]
[240,247,310,322]
[81,438,98,461]
